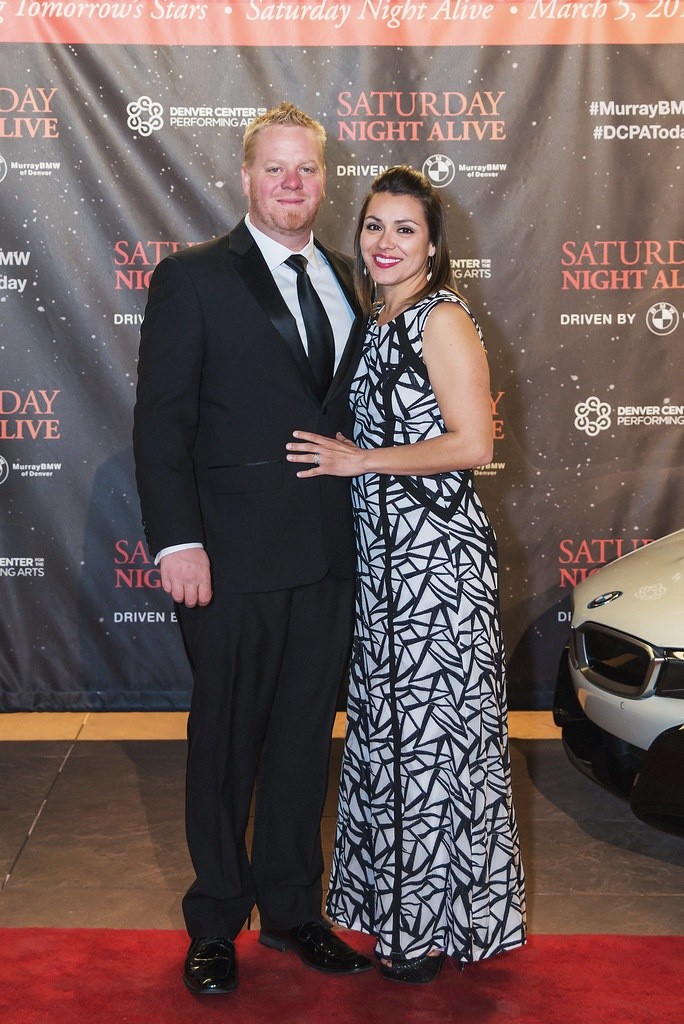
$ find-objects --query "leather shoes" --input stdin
[184,935,239,994]
[258,917,374,974]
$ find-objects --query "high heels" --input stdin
[381,952,466,984]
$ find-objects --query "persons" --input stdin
[283,166,529,985]
[132,99,378,996]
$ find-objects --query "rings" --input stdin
[314,453,320,464]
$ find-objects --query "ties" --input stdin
[284,254,336,401]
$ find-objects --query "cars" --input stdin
[552,530,684,831]
